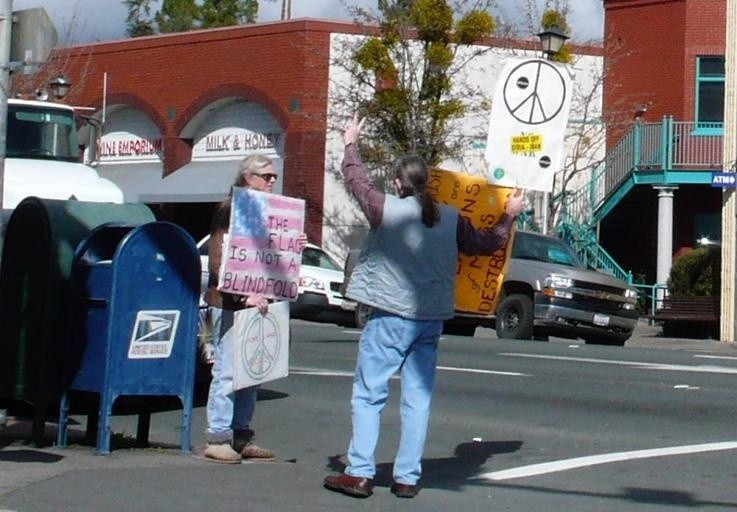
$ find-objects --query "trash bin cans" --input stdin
[60,222,203,453]
[1,197,160,441]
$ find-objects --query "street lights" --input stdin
[537,24,570,233]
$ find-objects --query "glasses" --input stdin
[255,173,277,181]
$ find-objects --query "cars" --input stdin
[189,228,356,327]
[440,227,641,347]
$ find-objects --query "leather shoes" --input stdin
[391,482,417,497]
[325,474,372,496]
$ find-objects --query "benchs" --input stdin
[651,295,720,340]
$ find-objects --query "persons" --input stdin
[203,153,309,464]
[324,109,526,497]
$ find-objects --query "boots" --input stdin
[203,429,276,464]
